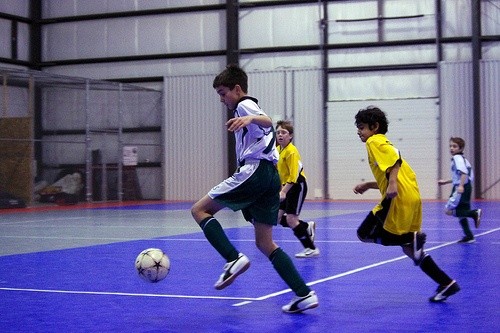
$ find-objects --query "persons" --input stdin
[191,65,318,313]
[438,137,481,243]
[353,108,461,302]
[274,120,320,258]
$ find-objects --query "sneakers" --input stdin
[306,221,315,242]
[282,291,319,313]
[428,279,461,302]
[411,231,427,265]
[295,247,320,257]
[213,252,251,290]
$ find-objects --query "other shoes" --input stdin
[473,209,482,229]
[457,236,476,243]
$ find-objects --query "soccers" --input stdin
[136,248,171,283]
